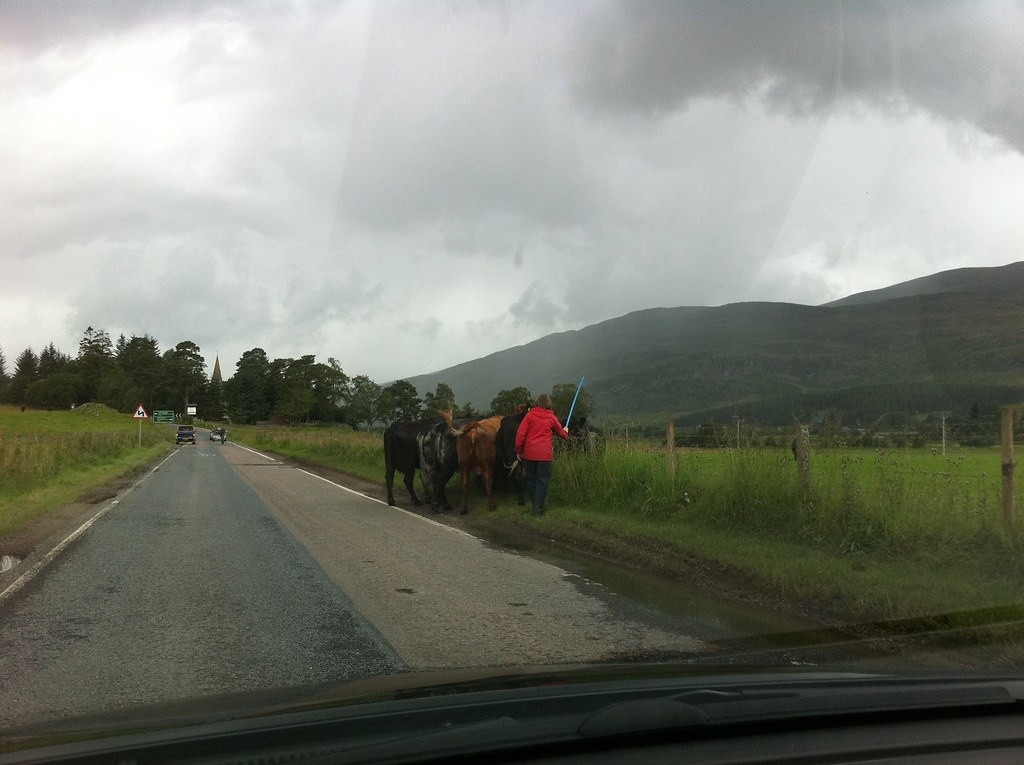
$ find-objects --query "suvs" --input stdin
[175,425,196,445]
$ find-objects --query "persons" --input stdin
[219,427,226,445]
[514,394,570,517]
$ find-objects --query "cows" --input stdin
[430,418,481,514]
[554,416,606,457]
[416,419,453,504]
[383,416,443,506]
[442,408,508,515]
[496,403,533,505]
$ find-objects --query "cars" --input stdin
[212,430,228,441]
[210,431,215,440]
